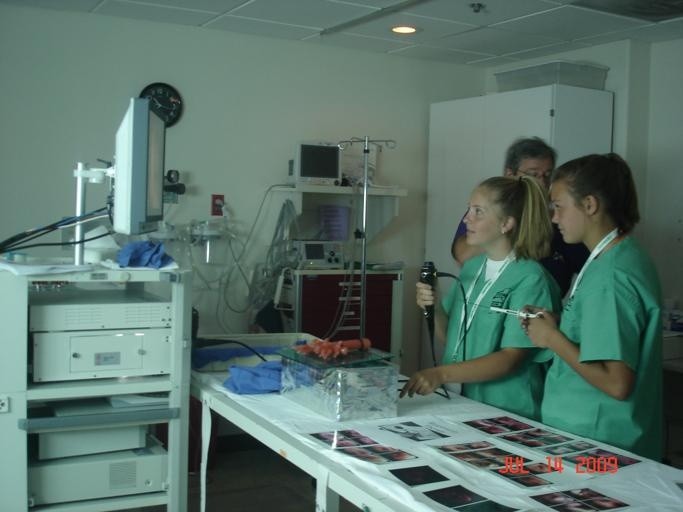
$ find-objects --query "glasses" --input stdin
[519,166,555,181]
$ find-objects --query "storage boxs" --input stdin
[494,59,610,91]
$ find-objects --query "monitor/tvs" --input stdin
[293,143,342,186]
[113,97,165,236]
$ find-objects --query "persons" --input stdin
[450,136,591,300]
[398,175,561,421]
[518,152,665,464]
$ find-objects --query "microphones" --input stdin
[420,262,437,336]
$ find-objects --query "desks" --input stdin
[190,333,683,512]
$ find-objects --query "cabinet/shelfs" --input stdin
[265,183,411,373]
[419,84,615,396]
[0,258,193,512]
